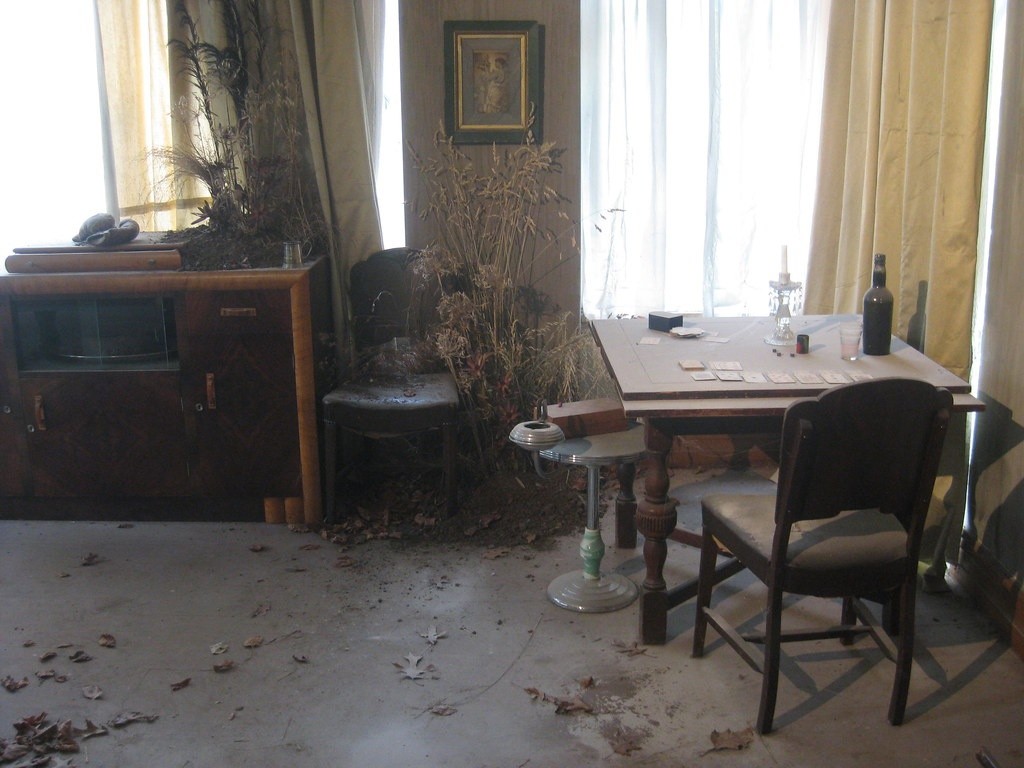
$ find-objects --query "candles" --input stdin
[780,245,787,275]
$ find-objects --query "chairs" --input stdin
[687,373,954,738]
[319,247,462,519]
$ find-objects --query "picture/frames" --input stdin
[441,20,543,145]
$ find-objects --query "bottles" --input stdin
[863,253,894,355]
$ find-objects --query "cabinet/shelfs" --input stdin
[0,249,330,528]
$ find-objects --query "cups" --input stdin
[838,321,863,361]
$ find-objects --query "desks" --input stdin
[590,312,984,645]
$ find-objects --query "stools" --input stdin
[538,418,648,612]
